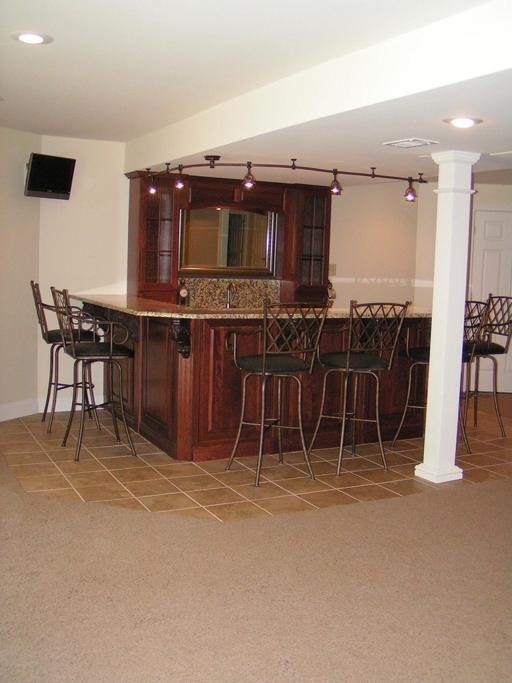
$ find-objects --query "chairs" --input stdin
[390,298,489,454]
[304,299,410,476]
[30,279,102,432]
[50,285,137,461]
[461,293,512,437]
[223,297,332,488]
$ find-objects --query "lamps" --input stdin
[241,161,257,191]
[174,163,185,189]
[403,176,418,202]
[329,168,344,195]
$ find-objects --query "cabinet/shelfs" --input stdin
[123,169,331,307]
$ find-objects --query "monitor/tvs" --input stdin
[25,152,75,199]
